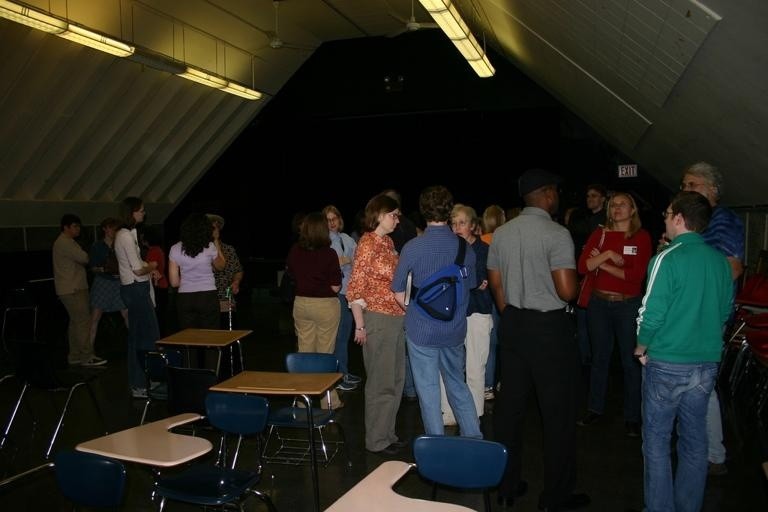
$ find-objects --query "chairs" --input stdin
[5,339,109,461]
[139,350,182,428]
[268,352,340,469]
[409,434,508,511]
[156,391,277,510]
[718,248,768,425]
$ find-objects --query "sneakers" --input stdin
[69,357,106,367]
[133,387,147,397]
[707,462,725,475]
[380,440,406,455]
[485,390,494,400]
[338,374,362,392]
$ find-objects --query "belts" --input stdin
[597,292,622,303]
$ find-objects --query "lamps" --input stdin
[0,0,263,101]
[418,0,496,79]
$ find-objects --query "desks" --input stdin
[204,369,344,510]
[155,328,254,400]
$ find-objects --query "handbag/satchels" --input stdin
[413,265,467,324]
[576,273,593,308]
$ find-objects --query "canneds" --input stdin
[225,287,233,298]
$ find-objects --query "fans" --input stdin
[253,1,317,57]
[384,0,440,36]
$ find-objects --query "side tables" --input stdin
[71,411,213,510]
[323,458,477,511]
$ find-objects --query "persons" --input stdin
[52,195,244,398]
[288,186,507,455]
[566,164,746,510]
[484,167,593,510]
